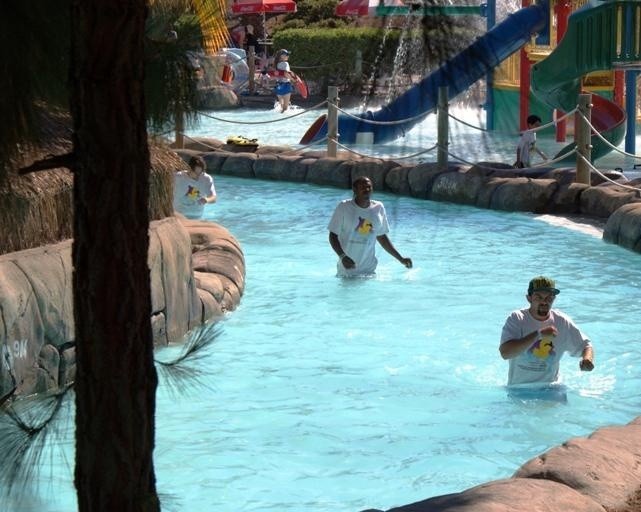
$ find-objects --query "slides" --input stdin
[529,1,627,167]
[299,5,546,145]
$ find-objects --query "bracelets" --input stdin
[339,252,346,259]
[540,152,544,155]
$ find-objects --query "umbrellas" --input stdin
[231,1,298,55]
[336,0,487,17]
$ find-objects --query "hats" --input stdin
[529,276,560,295]
[280,50,292,56]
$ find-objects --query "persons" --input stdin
[171,153,218,220]
[498,276,596,392]
[513,114,549,169]
[230,25,255,52]
[325,175,414,278]
[274,49,297,113]
